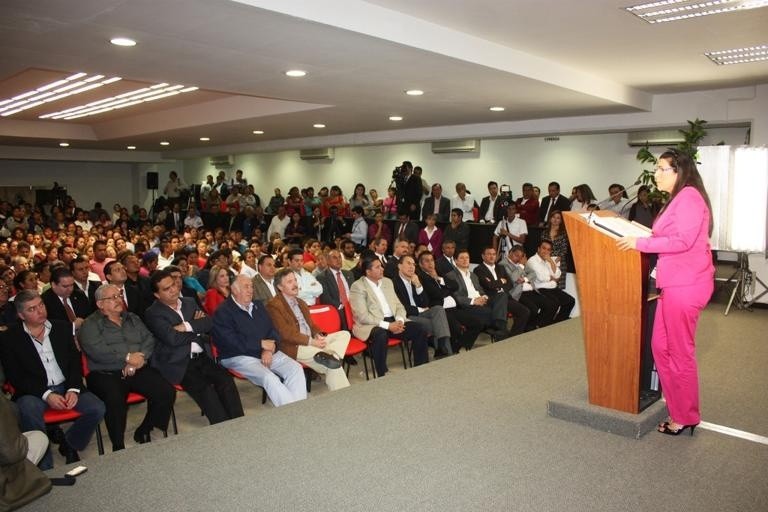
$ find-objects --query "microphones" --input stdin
[579,178,641,221]
[617,184,653,218]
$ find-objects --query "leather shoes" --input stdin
[59,443,80,464]
[134,427,152,444]
[312,352,342,369]
[434,348,451,360]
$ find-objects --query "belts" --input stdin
[189,351,207,358]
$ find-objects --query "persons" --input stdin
[615,147,716,436]
[1,162,656,471]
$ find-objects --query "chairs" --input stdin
[0,291,514,468]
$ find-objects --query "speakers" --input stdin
[146,171,159,191]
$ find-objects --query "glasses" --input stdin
[97,294,124,300]
[654,164,676,173]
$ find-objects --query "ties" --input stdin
[399,223,406,234]
[547,198,556,220]
[381,256,388,265]
[336,272,348,304]
[175,214,179,229]
[62,296,78,322]
[449,257,456,266]
[120,289,128,310]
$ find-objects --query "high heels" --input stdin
[657,417,701,437]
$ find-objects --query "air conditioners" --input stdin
[208,153,234,166]
[430,137,480,154]
[624,127,692,146]
[298,146,334,161]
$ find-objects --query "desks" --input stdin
[162,209,574,274]
